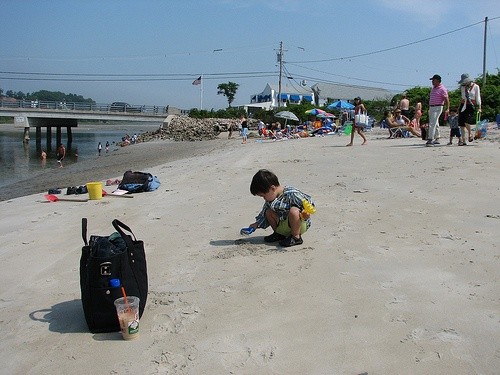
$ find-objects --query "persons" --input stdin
[122,108,351,143]
[42,143,65,168]
[98,142,109,156]
[249,169,314,247]
[31,99,66,108]
[381,93,421,138]
[426,75,481,145]
[347,96,367,147]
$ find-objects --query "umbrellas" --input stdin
[316,112,335,121]
[328,100,355,117]
[305,108,326,122]
[274,111,299,130]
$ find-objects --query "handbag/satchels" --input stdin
[475,112,487,138]
[123,171,149,185]
[354,108,369,128]
[80,218,148,334]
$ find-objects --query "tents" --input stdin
[311,82,404,107]
[251,82,313,103]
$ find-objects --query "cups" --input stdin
[114,295,140,340]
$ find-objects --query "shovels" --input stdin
[44,194,88,203]
[102,190,134,199]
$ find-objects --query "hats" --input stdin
[429,75,441,82]
[458,74,470,85]
[354,97,360,100]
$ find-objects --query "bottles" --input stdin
[111,279,120,287]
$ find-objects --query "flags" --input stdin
[192,76,201,85]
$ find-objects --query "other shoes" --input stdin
[425,140,434,147]
[448,142,452,145]
[264,232,285,243]
[279,235,303,247]
[469,136,474,142]
[458,141,463,145]
[434,139,439,143]
[463,139,467,145]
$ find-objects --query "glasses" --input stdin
[397,111,401,113]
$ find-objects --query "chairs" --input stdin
[380,120,409,139]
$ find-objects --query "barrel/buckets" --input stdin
[85,181,102,200]
